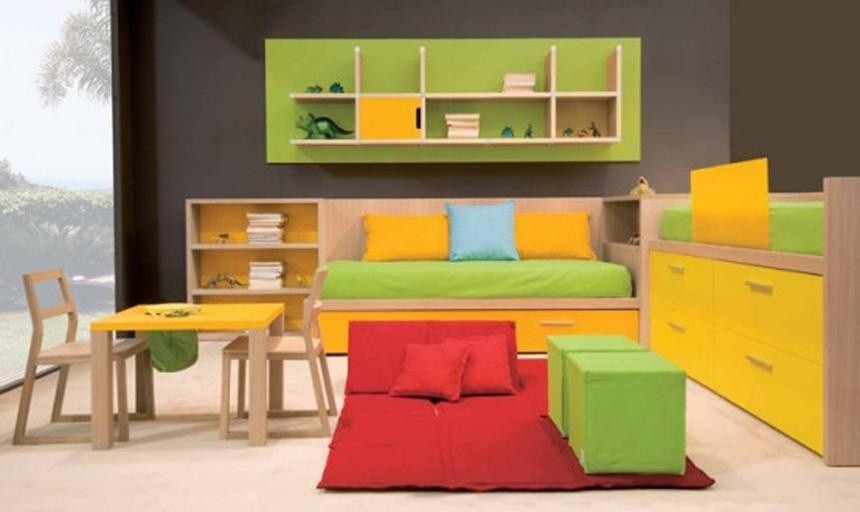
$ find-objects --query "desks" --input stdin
[89,302,284,451]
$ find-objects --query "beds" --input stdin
[315,197,641,354]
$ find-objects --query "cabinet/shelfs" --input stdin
[186,198,323,341]
[289,46,621,145]
[650,175,860,467]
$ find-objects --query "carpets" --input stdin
[316,357,716,492]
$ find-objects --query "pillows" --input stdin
[439,333,518,397]
[359,209,598,260]
[389,341,474,401]
[444,198,519,261]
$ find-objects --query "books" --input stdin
[500,71,537,90]
[248,257,285,289]
[244,210,288,244]
[443,112,480,137]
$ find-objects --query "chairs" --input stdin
[13,271,154,445]
[218,265,336,439]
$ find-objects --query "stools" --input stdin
[566,350,686,474]
[545,333,646,437]
